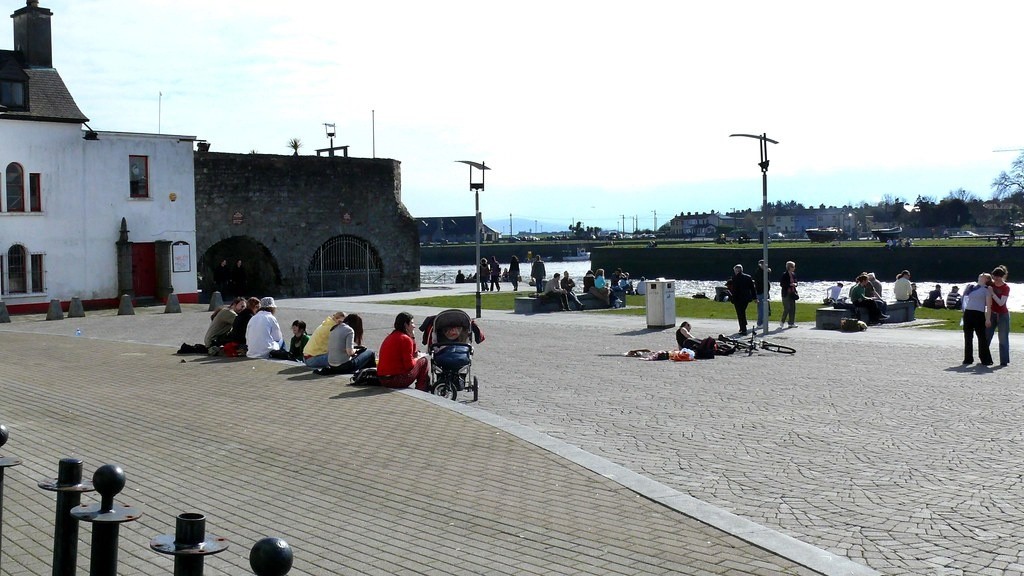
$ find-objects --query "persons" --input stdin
[849,272,890,324]
[611,268,629,289]
[531,255,546,293]
[987,265,1010,366]
[456,270,465,283]
[997,235,1002,245]
[894,270,921,309]
[647,240,657,248]
[779,261,799,328]
[545,271,586,310]
[306,311,376,373]
[675,322,694,350]
[289,320,310,361]
[726,260,771,335]
[583,269,606,292]
[886,237,910,247]
[963,273,993,365]
[827,283,844,301]
[204,297,287,359]
[480,255,520,291]
[634,276,646,295]
[929,285,942,300]
[377,312,430,391]
[946,286,963,309]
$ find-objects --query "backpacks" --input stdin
[695,337,716,358]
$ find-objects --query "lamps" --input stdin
[178,138,211,154]
[82,123,100,141]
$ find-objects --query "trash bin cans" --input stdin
[645,278,676,328]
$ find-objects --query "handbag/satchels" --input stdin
[529,279,536,286]
[674,347,695,360]
[269,350,289,358]
[519,275,522,282]
[350,367,381,386]
[795,291,799,300]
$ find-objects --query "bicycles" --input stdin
[712,325,796,355]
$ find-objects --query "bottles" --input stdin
[375,354,379,367]
[76,328,80,336]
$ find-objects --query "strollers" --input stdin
[427,309,479,401]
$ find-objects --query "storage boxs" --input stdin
[658,353,669,360]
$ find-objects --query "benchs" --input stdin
[514,293,626,315]
[815,300,914,331]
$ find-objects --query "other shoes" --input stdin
[788,324,798,327]
[870,322,881,325]
[757,325,769,328]
[780,321,783,327]
[879,314,890,320]
[565,306,572,310]
[559,304,566,311]
[738,332,747,335]
[578,304,588,310]
[615,298,623,303]
[1000,363,1007,366]
[613,305,620,308]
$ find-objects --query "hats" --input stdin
[247,297,262,307]
[259,297,277,307]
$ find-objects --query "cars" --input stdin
[959,231,974,236]
[508,231,656,242]
[770,232,785,239]
